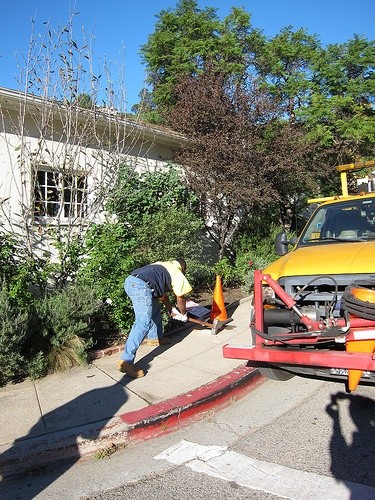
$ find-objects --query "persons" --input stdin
[118,258,192,377]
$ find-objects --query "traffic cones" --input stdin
[341,286,375,392]
[207,275,231,325]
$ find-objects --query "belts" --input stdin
[130,272,154,289]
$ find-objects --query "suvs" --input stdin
[222,159,375,384]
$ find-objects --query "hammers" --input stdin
[171,312,220,336]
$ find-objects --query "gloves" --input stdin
[171,307,188,322]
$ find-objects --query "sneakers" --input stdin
[116,360,145,378]
[147,338,171,346]
